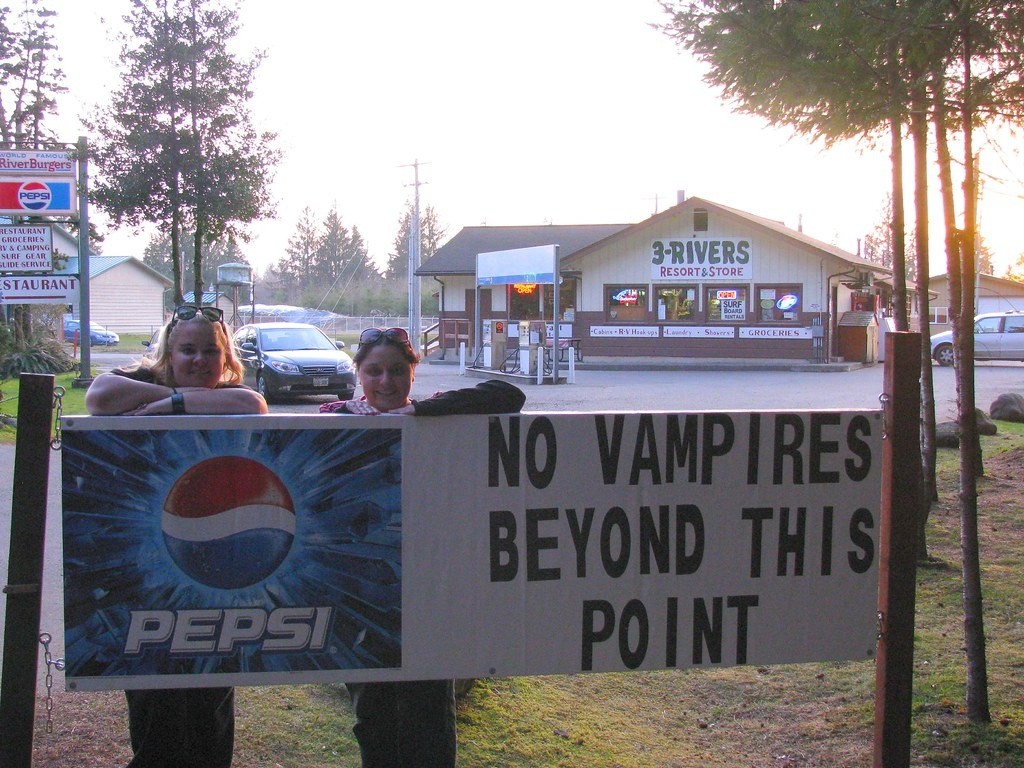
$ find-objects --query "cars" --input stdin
[143,329,163,360]
[231,322,357,404]
[64,319,119,347]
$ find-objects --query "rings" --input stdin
[137,403,145,409]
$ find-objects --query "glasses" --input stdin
[172,305,224,325]
[358,328,411,347]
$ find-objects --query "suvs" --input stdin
[930,310,1024,367]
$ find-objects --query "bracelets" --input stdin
[171,386,177,394]
[172,394,184,414]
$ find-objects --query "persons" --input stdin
[314,328,526,767]
[85,305,269,768]
